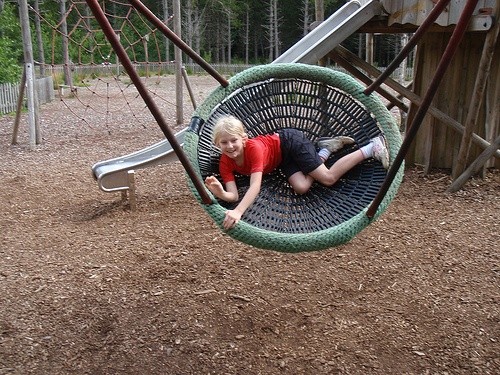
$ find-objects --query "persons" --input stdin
[202,114,391,231]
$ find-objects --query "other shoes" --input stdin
[369,133,389,169]
[317,135,355,152]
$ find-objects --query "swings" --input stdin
[87,0,479,253]
